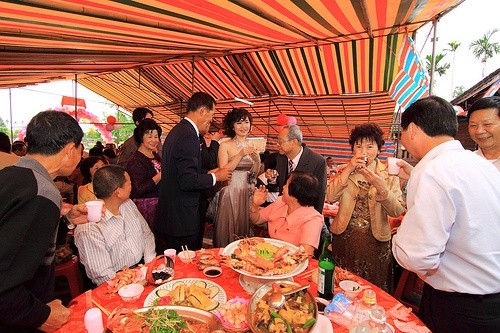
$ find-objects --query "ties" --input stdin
[288,160,294,172]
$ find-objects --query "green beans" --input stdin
[269,310,316,332]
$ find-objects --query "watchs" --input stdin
[62,215,77,230]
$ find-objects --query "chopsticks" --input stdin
[181,244,193,265]
[91,299,111,316]
[296,267,318,279]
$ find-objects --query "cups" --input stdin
[201,238,214,260]
[86,201,102,223]
[84,308,104,333]
[387,157,402,176]
[164,249,176,265]
[268,169,277,184]
[358,156,368,170]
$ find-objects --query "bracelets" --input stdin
[249,206,260,214]
[377,188,390,195]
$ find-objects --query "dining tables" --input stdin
[51,248,431,333]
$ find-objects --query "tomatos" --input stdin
[157,295,172,306]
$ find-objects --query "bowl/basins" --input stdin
[118,284,144,302]
[178,250,195,264]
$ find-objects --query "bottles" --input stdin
[356,305,392,333]
[316,233,336,300]
[350,288,378,329]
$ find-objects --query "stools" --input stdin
[52,255,84,305]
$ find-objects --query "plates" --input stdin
[223,237,308,280]
[149,267,175,285]
[119,305,222,333]
[238,273,294,294]
[339,280,362,293]
[203,267,222,278]
[249,280,318,333]
[312,314,334,333]
[142,278,227,315]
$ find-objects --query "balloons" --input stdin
[17,107,122,142]
[276,114,297,126]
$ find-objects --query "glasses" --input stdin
[276,139,294,145]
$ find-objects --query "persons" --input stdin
[0,133,27,170]
[199,130,225,249]
[468,96,500,172]
[56,107,163,232]
[248,170,325,260]
[326,122,405,290]
[73,164,155,288]
[265,125,328,213]
[0,110,107,333]
[156,92,233,256]
[390,96,500,333]
[214,107,261,247]
[325,156,338,175]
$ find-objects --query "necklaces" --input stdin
[233,135,249,147]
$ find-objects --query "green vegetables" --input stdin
[137,308,187,333]
[255,248,273,259]
